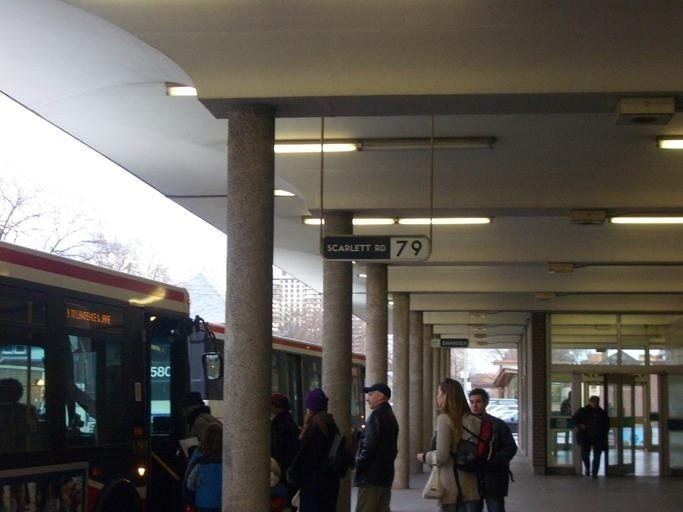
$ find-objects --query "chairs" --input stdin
[0,377,38,451]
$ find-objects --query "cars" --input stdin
[483,397,519,431]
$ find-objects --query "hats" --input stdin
[271,393,291,412]
[305,389,329,412]
[182,392,204,408]
[364,383,391,400]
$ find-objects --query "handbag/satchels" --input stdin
[423,450,444,499]
[328,434,349,479]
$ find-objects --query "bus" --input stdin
[41,317,365,460]
[0,240,222,510]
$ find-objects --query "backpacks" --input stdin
[431,412,495,474]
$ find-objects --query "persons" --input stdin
[269,392,302,511]
[285,387,342,512]
[468,388,517,511]
[416,377,485,511]
[352,382,400,511]
[559,391,572,444]
[182,423,223,512]
[174,390,211,471]
[570,395,610,478]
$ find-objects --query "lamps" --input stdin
[657,134,681,150]
[359,134,496,151]
[397,216,492,225]
[604,209,681,223]
[163,84,196,98]
[299,213,397,225]
[273,137,359,154]
[272,187,294,198]
